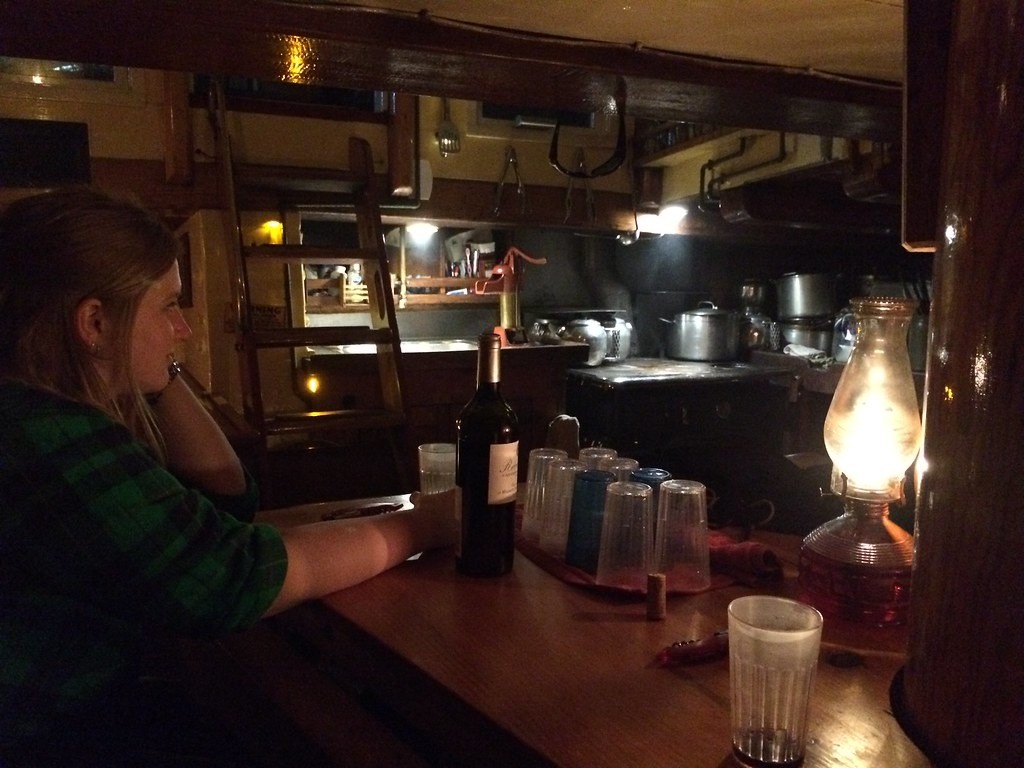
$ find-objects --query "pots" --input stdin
[656,300,744,364]
[773,272,840,319]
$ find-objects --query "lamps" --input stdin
[795,292,939,632]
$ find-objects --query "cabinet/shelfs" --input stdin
[297,217,519,315]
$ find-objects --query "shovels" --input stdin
[438,97,462,155]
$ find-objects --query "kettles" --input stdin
[601,315,633,364]
[560,316,621,368]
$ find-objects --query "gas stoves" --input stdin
[566,357,789,445]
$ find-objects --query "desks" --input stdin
[563,355,796,490]
[249,483,938,767]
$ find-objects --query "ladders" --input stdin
[211,127,420,507]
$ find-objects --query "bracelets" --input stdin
[142,359,182,406]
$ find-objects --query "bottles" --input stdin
[457,330,522,580]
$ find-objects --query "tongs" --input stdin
[566,148,597,223]
[495,147,525,217]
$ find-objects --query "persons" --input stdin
[1,190,459,767]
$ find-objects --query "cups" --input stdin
[726,593,826,768]
[526,447,709,593]
[417,442,460,497]
